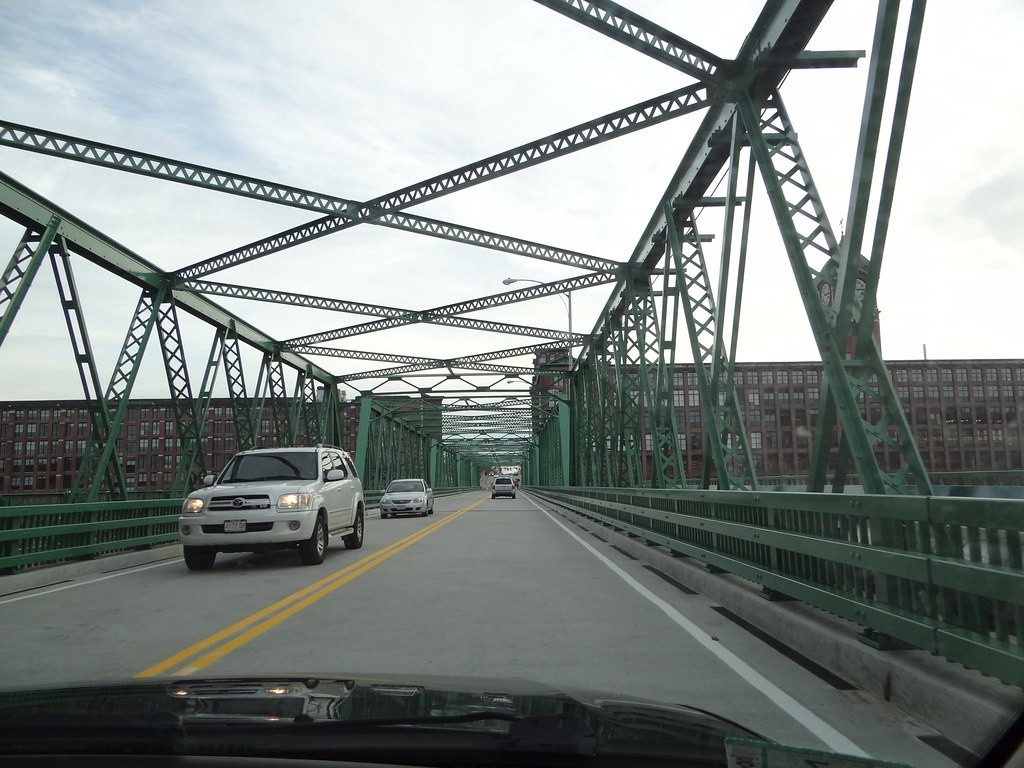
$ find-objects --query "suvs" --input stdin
[175,443,367,572]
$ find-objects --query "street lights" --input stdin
[502,278,574,390]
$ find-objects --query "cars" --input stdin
[490,477,517,500]
[378,478,434,519]
